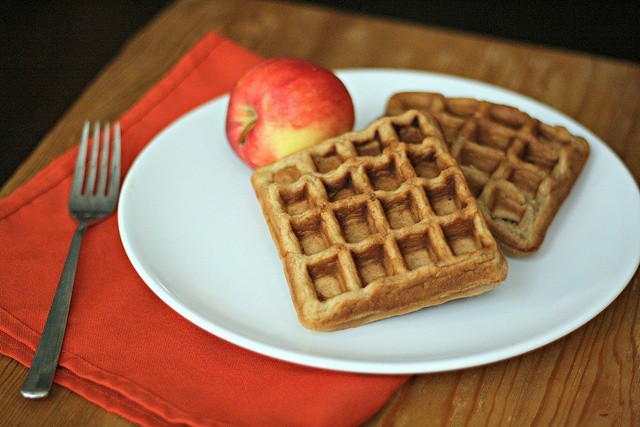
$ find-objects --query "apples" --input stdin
[226,58,354,168]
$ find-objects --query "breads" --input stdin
[251,108,508,332]
[388,92,589,256]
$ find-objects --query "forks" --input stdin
[18,119,124,401]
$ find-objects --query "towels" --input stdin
[1,31,413,426]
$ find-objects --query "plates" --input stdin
[117,67,637,375]
